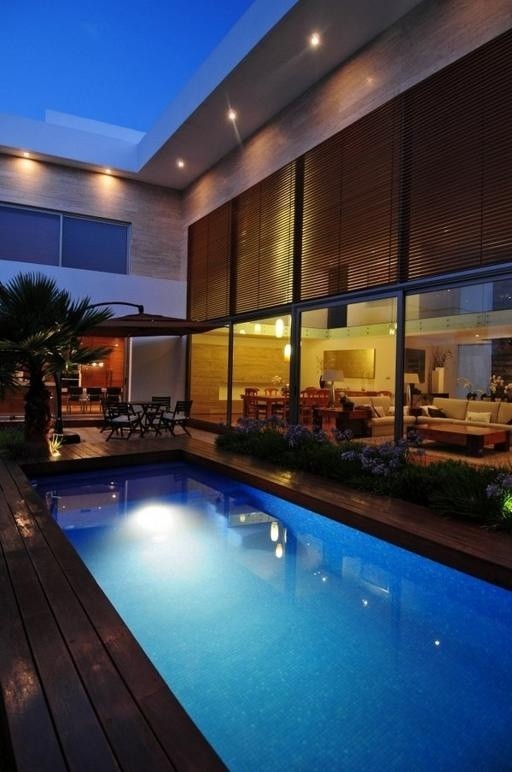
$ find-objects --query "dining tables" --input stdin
[482,393,492,401]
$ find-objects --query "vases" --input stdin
[457,377,473,389]
[488,374,505,392]
[504,383,512,395]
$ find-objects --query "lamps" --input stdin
[66,387,193,442]
[243,388,391,427]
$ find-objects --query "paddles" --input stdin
[351,434,512,471]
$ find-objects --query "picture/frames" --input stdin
[314,406,372,440]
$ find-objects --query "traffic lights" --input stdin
[466,412,493,424]
[429,407,448,417]
[365,404,410,418]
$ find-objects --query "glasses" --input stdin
[404,373,420,407]
[275,317,285,339]
[284,342,292,363]
[320,370,345,407]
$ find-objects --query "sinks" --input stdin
[415,397,512,446]
[347,396,416,437]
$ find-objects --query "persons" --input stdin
[340,391,378,418]
